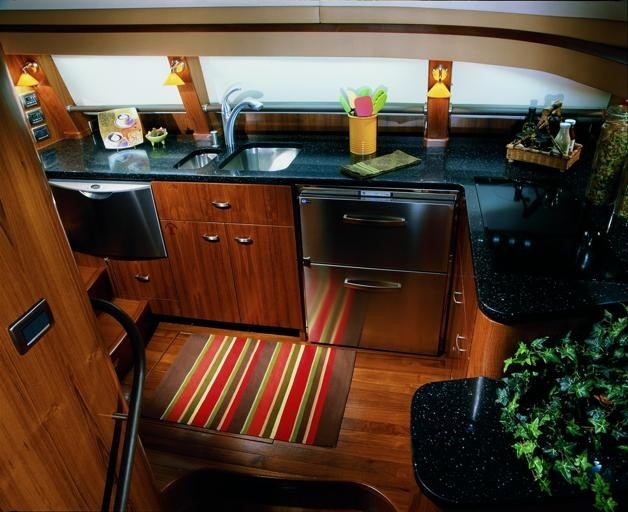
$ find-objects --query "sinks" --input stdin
[218,141,305,172]
[172,148,219,170]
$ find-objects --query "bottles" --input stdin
[584,105,628,221]
[521,100,575,157]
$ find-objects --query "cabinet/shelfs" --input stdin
[445,207,518,380]
[301,188,458,357]
[102,181,304,341]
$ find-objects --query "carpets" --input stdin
[139,333,358,449]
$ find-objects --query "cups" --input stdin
[108,132,124,144]
[347,112,378,157]
[117,115,131,124]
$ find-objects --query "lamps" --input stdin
[164,59,186,86]
[15,62,39,87]
[426,64,452,99]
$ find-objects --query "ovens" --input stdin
[294,191,455,357]
[47,179,168,262]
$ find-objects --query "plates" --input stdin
[113,120,136,127]
[104,139,128,149]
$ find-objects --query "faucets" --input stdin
[219,87,264,150]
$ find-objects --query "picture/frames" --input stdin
[18,92,52,143]
